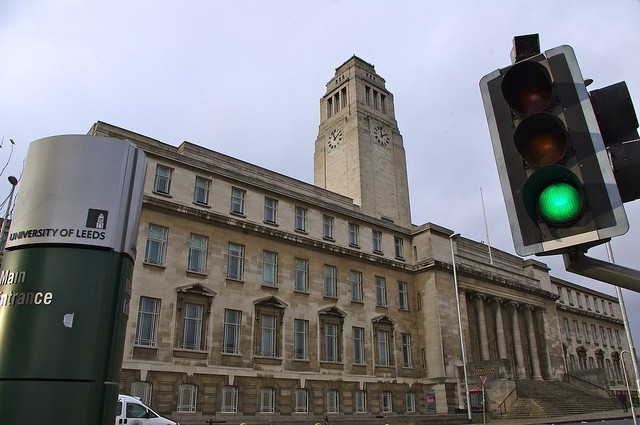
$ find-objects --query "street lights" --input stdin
[0,175,18,246]
[449,232,473,424]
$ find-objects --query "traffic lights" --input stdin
[479,46,629,256]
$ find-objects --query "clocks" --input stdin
[325,127,344,149]
[373,123,391,149]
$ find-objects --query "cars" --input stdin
[114,394,181,425]
